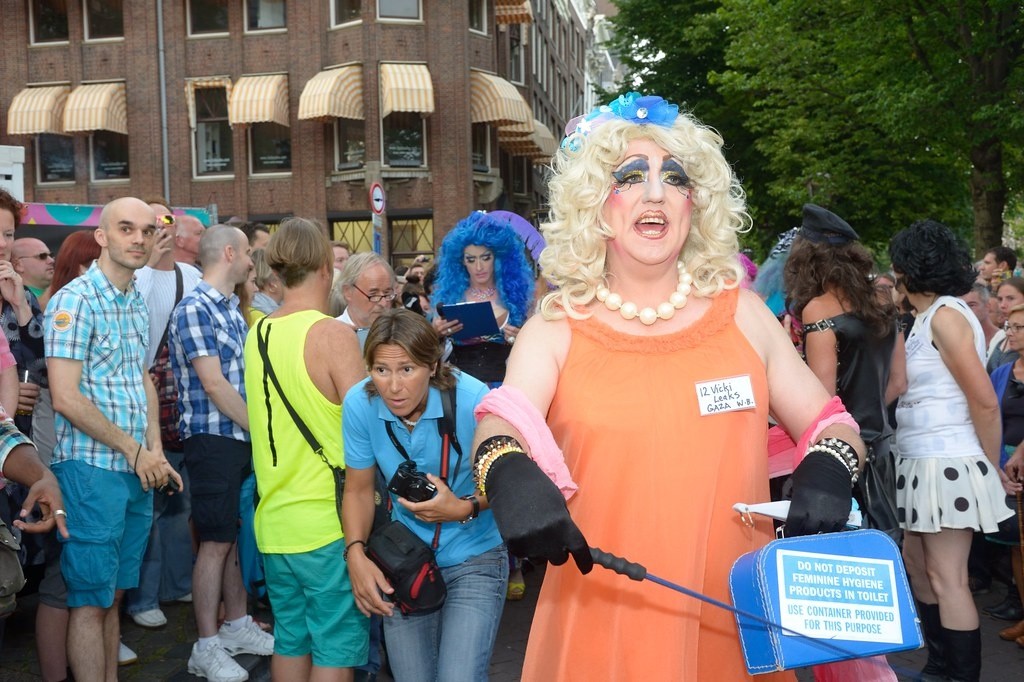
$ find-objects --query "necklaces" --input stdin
[470,287,496,299]
[595,259,693,325]
[400,417,416,427]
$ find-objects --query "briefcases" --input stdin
[728,518,923,676]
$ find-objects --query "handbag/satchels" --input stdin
[331,466,389,531]
[0,524,26,620]
[366,519,447,617]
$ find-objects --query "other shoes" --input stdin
[1016,636,1024,646]
[119,642,138,664]
[131,609,167,628]
[1000,621,1024,640]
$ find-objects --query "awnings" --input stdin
[470,72,559,164]
[184,63,436,128]
[8,83,129,136]
[494,0,534,24]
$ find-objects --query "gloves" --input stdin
[473,435,594,575]
[781,438,862,539]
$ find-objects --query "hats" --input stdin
[800,202,857,245]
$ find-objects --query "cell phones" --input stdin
[997,271,1011,281]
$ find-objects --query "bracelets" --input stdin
[460,495,480,524]
[473,437,527,496]
[344,540,368,561]
[806,438,858,488]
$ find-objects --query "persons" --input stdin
[471,91,897,682]
[0,187,550,682]
[739,202,1024,682]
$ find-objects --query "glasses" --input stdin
[18,252,56,260]
[157,214,178,229]
[1003,324,1023,332]
[351,283,397,303]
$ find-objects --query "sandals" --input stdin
[506,583,526,600]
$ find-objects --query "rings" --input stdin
[508,337,515,343]
[448,328,453,334]
[55,510,67,517]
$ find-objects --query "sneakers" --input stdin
[186,642,249,682]
[220,617,274,655]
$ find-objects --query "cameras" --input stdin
[157,476,179,495]
[387,461,437,503]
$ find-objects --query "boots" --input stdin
[914,598,940,682]
[939,626,983,682]
[981,581,1014,615]
[991,585,1024,620]
